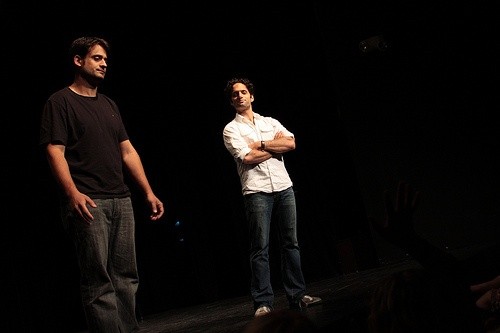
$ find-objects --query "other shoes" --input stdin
[254,306,271,318]
[289,295,322,308]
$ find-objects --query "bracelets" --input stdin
[261,140,265,151]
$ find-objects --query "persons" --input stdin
[223,79,321,317]
[42,37,164,333]
